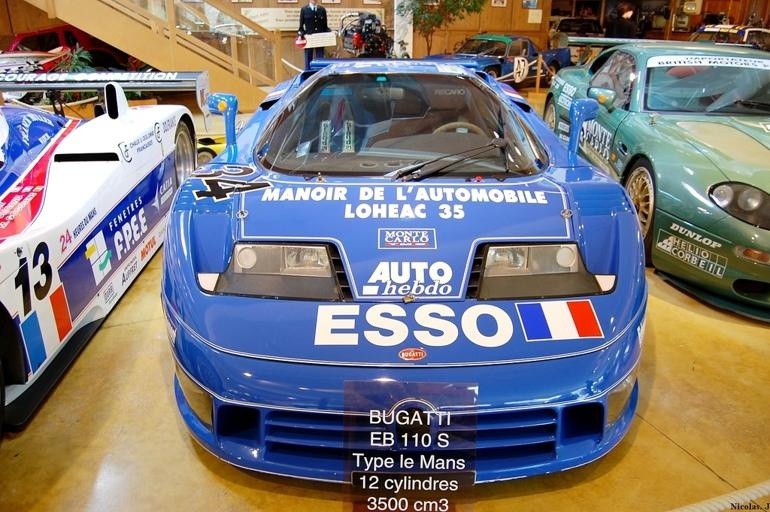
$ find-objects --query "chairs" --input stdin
[410,81,472,134]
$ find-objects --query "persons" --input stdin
[298,0,331,71]
[602,2,636,50]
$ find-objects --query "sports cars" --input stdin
[0,82,198,432]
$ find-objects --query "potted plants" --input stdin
[100,68,159,106]
[33,42,98,120]
